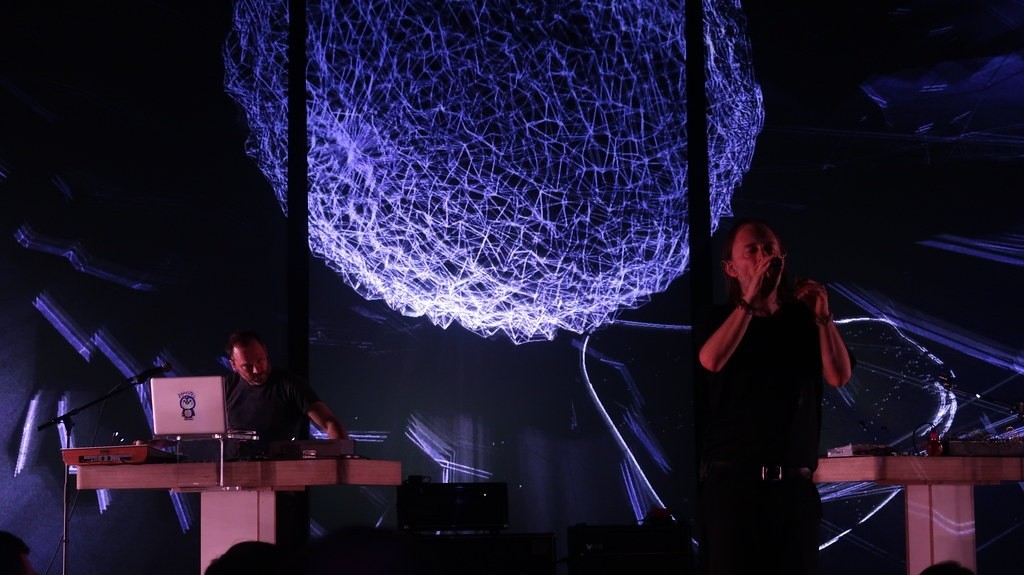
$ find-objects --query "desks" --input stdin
[812,455,1024,575]
[77,460,402,575]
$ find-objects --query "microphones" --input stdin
[768,257,782,276]
[132,362,173,381]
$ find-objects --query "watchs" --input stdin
[815,312,834,327]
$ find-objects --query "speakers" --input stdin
[396,480,508,534]
[424,530,556,574]
[567,520,695,575]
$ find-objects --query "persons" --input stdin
[0,529,41,575]
[691,219,855,575]
[224,328,349,439]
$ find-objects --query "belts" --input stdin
[698,457,814,485]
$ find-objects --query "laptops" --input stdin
[151,375,256,434]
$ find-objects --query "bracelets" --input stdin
[735,295,757,314]
